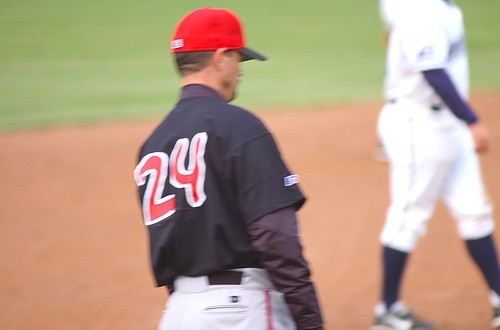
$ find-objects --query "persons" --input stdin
[131,7,324,330]
[371,0,500,330]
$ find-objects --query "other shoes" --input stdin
[491,307,500,327]
[373,309,438,330]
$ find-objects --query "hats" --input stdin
[170,8,267,62]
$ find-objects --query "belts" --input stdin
[168,270,242,294]
[387,99,442,111]
